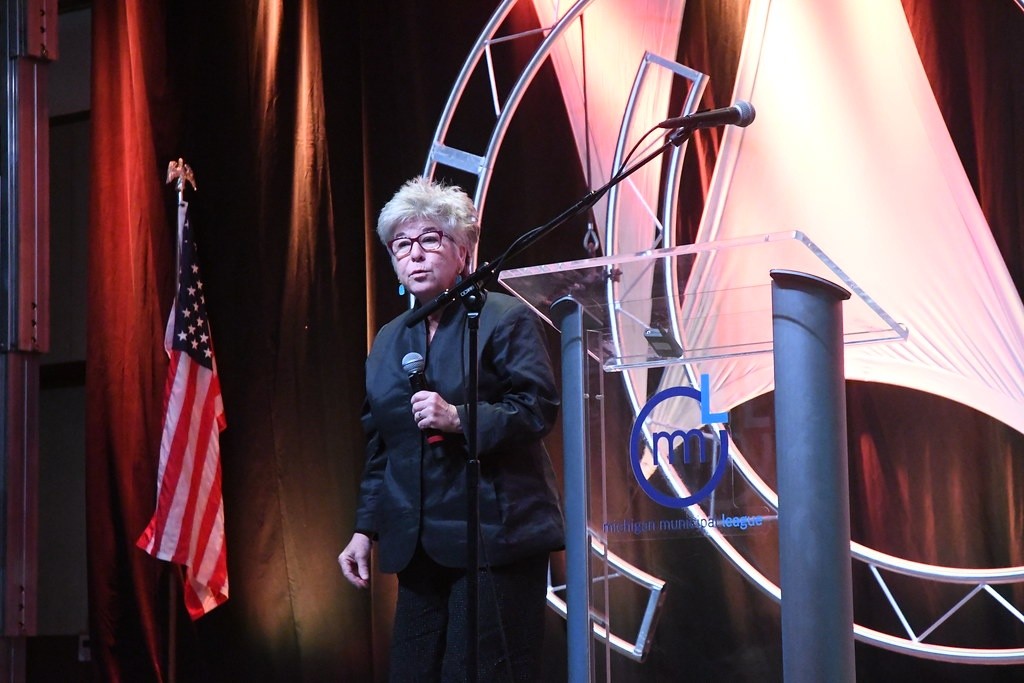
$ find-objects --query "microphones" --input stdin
[403,352,444,446]
[659,100,756,129]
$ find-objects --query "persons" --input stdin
[338,176,567,683]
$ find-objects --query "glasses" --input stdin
[387,230,456,259]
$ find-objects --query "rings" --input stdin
[418,411,422,419]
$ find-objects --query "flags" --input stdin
[134,200,229,622]
